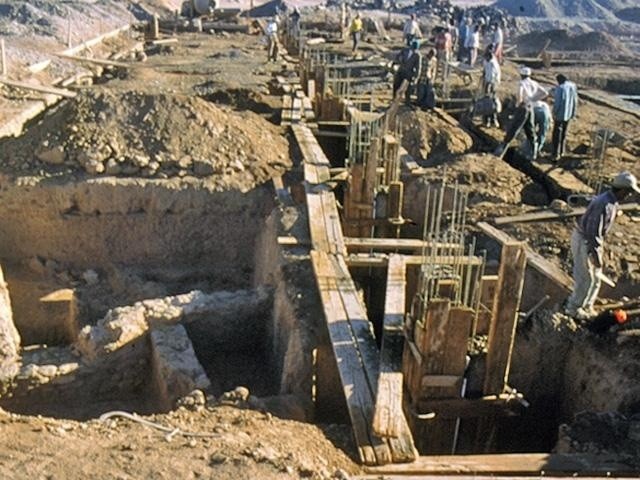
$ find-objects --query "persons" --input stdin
[390,13,578,161]
[564,172,640,319]
[264,15,282,64]
[349,10,364,54]
[288,5,300,37]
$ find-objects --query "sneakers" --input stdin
[564,304,598,320]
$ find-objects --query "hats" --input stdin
[412,41,420,49]
[272,14,282,24]
[520,68,531,75]
[602,170,640,195]
[477,17,485,24]
